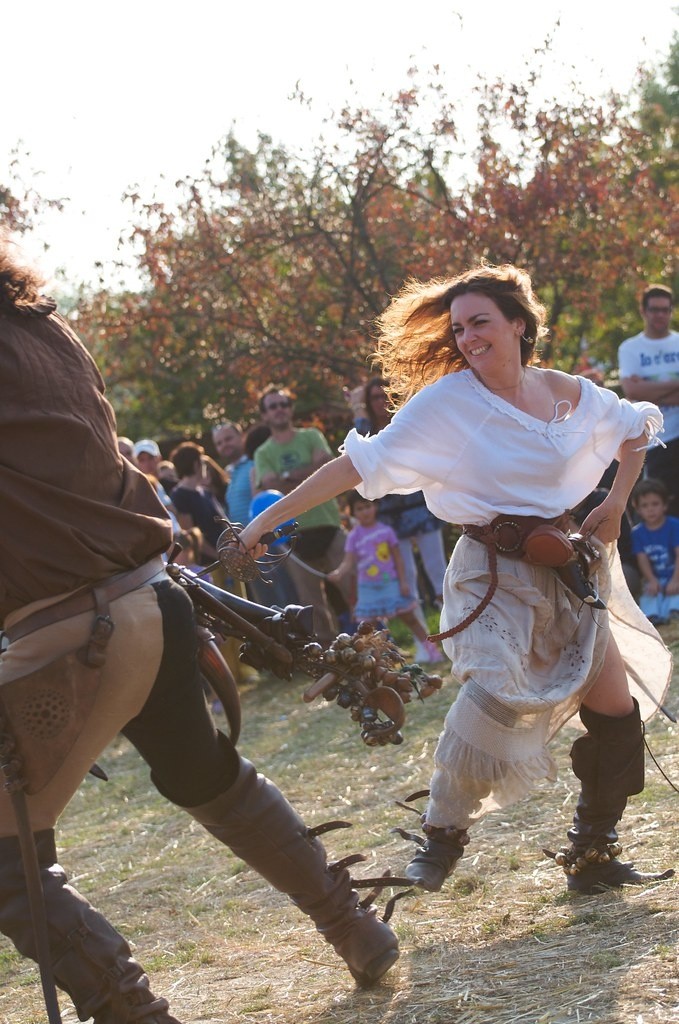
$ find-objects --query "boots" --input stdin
[149,730,400,989]
[558,697,675,893]
[1,829,182,1023]
[403,823,472,894]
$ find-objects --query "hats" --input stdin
[134,440,160,459]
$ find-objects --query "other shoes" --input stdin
[421,641,444,664]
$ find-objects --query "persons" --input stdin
[230,263,674,895]
[0,259,397,1024]
[571,283,679,626]
[116,365,458,715]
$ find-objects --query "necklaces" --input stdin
[486,364,526,390]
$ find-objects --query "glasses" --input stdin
[265,401,289,411]
[646,305,672,313]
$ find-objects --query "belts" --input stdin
[0,553,167,671]
